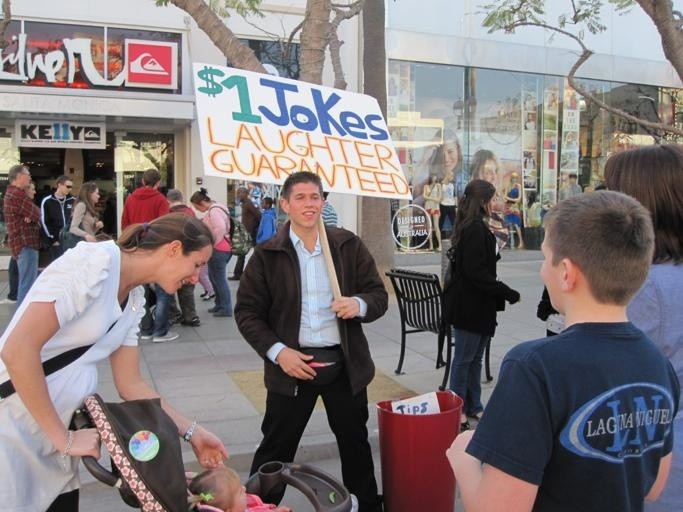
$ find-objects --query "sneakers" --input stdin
[170,316,201,328]
[200,291,233,318]
[138,330,182,344]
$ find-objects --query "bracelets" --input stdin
[60,430,73,457]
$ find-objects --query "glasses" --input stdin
[66,185,73,189]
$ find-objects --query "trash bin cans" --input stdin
[377,389,464,512]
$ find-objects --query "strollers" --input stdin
[67,386,354,511]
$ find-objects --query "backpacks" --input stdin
[208,206,253,256]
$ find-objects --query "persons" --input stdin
[2,165,338,344]
[410,128,525,251]
[559,174,582,202]
[0,211,228,512]
[604,143,683,512]
[441,178,520,433]
[233,171,388,512]
[189,468,290,512]
[445,190,681,512]
[536,284,566,337]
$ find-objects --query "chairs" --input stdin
[384,268,493,393]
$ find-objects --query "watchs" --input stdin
[184,421,197,441]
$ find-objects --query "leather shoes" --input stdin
[459,414,470,431]
